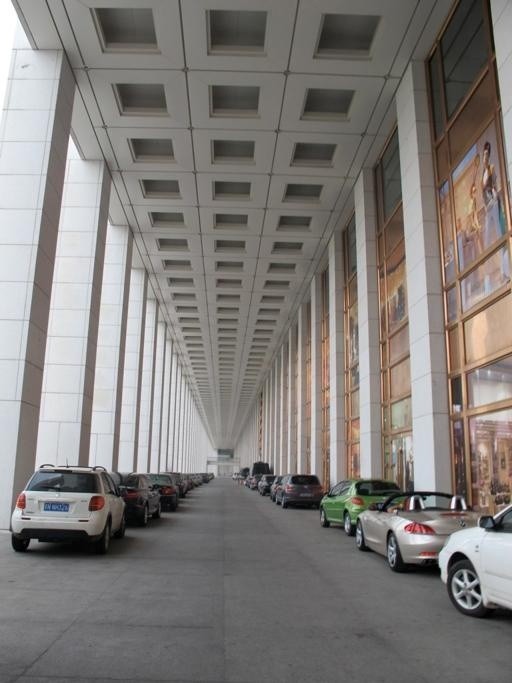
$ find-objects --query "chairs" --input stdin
[403,495,424,511]
[449,495,468,512]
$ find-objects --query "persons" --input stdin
[447,141,510,323]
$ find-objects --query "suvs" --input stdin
[10,462,124,557]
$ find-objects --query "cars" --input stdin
[232,462,323,508]
[148,469,214,512]
[354,490,485,571]
[320,478,406,536]
[109,471,161,528]
[436,503,511,618]
